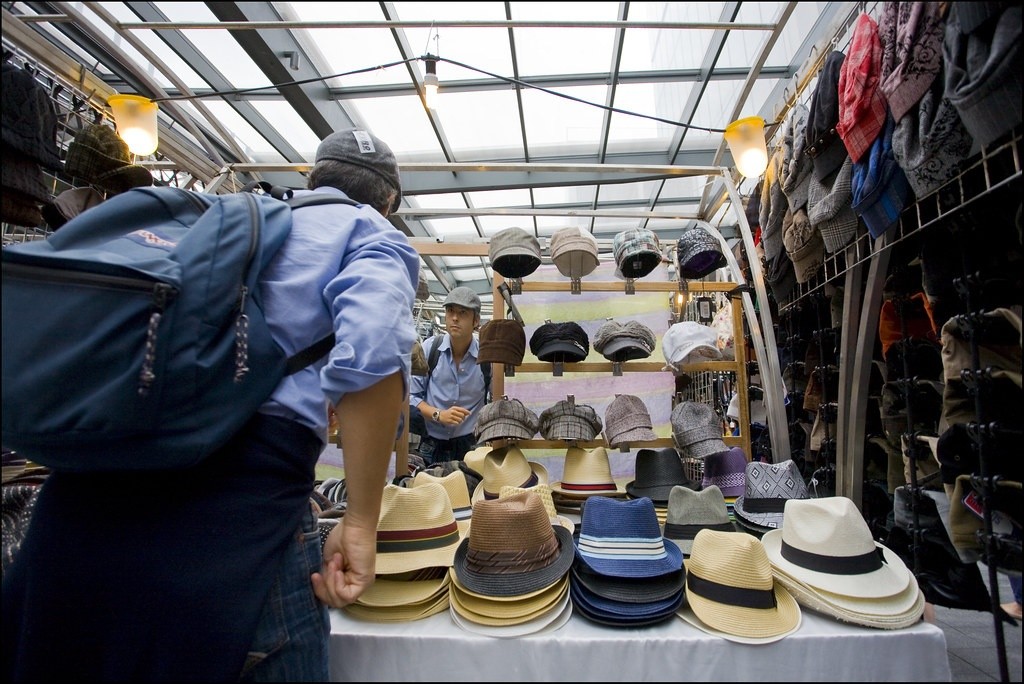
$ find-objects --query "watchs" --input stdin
[431,409,441,424]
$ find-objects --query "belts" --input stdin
[423,433,475,451]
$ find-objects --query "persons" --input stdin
[406,286,490,462]
[1,128,420,683]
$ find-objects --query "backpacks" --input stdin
[0,180,360,493]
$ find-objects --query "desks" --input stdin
[325,605,952,682]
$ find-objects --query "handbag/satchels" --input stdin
[412,338,430,376]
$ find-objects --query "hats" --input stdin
[662,321,723,371]
[0,63,62,170]
[488,227,541,278]
[0,186,41,227]
[475,320,526,367]
[63,123,155,193]
[315,128,401,212]
[727,0,1024,632]
[612,229,664,280]
[441,287,482,312]
[593,316,656,363]
[678,229,728,278]
[311,395,923,644]
[41,187,105,229]
[550,228,599,278]
[0,140,54,208]
[530,319,590,363]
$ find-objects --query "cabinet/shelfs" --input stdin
[491,268,754,464]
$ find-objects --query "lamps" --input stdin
[724,116,769,179]
[107,93,159,157]
